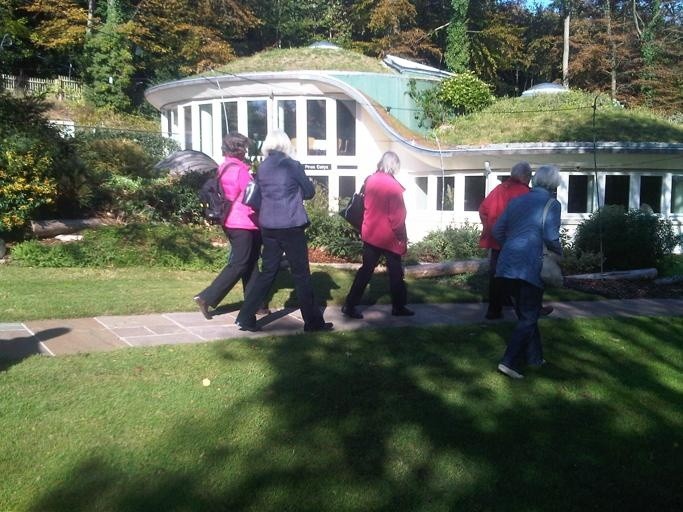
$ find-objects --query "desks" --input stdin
[314,139,326,155]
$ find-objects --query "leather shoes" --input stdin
[391,307,416,316]
[341,305,364,319]
[255,307,271,315]
[193,293,213,320]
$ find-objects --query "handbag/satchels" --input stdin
[241,180,261,212]
[339,193,365,231]
[539,249,566,289]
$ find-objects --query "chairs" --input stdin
[290,136,314,154]
[339,138,348,155]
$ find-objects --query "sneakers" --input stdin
[303,322,334,332]
[539,359,551,367]
[497,363,526,380]
[538,305,553,318]
[485,313,504,320]
[235,319,258,331]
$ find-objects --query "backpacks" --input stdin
[197,176,231,226]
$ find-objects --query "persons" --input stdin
[492,165,565,380]
[234,129,335,333]
[192,129,272,320]
[340,150,415,319]
[477,161,554,322]
